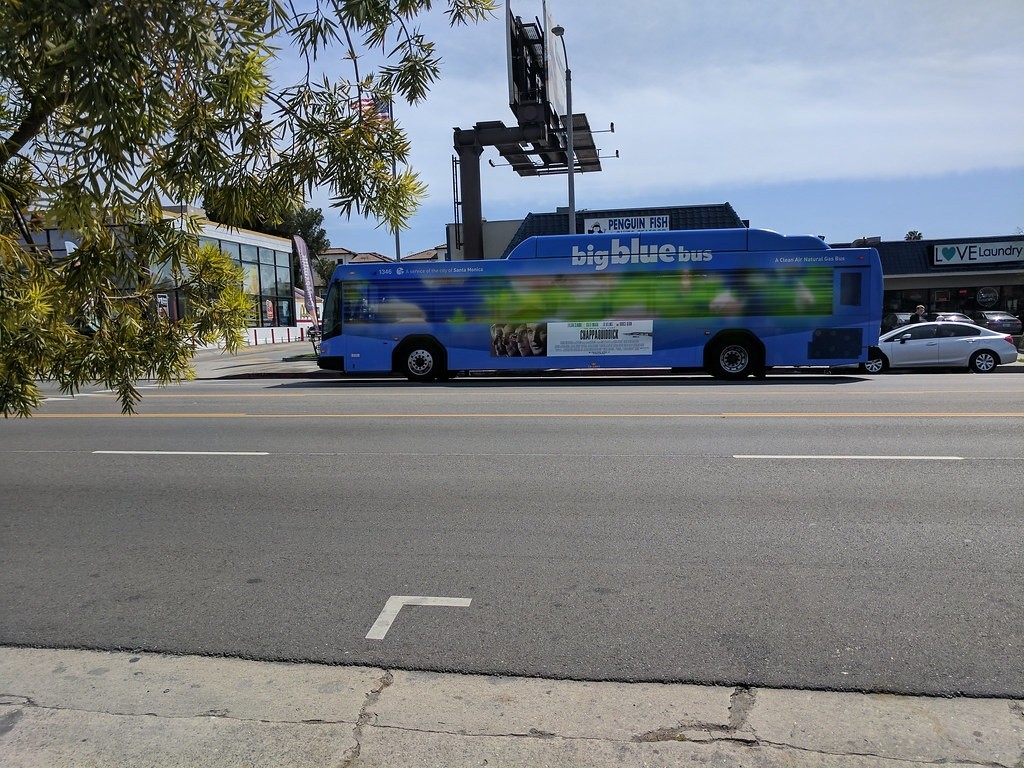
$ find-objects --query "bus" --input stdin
[316,227,883,383]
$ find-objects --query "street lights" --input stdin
[551,25,577,235]
[375,74,400,262]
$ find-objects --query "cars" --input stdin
[967,311,1023,349]
[830,321,1018,375]
[881,313,928,335]
[922,312,975,325]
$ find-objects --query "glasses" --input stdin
[511,331,528,342]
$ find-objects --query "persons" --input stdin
[490,323,547,356]
[910,305,925,323]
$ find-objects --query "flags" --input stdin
[351,96,390,123]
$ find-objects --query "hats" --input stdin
[935,316,944,322]
